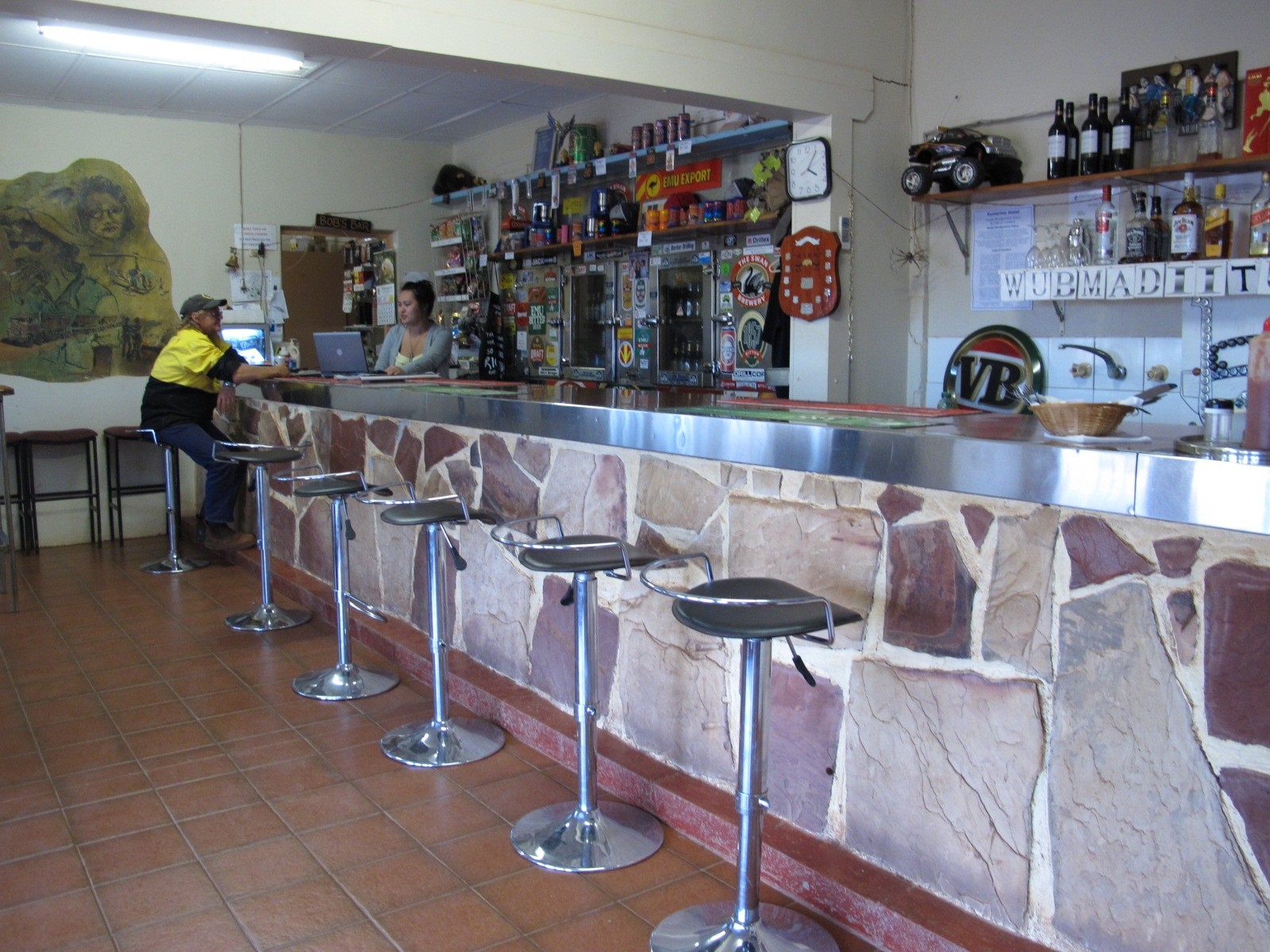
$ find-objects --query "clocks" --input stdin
[784,136,833,202]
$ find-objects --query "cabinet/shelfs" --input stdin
[430,237,469,303]
[430,119,793,263]
[914,153,1270,336]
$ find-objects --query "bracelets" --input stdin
[223,380,237,387]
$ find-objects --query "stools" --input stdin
[354,478,508,768]
[104,424,182,548]
[272,463,399,702]
[640,549,863,952]
[124,427,209,575]
[0,428,102,557]
[210,440,314,633]
[492,513,670,878]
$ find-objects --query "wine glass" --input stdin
[1024,220,1091,269]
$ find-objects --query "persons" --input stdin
[140,294,289,551]
[372,280,453,379]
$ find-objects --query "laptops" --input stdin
[313,331,388,377]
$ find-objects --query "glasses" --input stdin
[205,311,223,317]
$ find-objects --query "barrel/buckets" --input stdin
[569,123,597,163]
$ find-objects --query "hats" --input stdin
[180,294,227,319]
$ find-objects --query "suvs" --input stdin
[900,125,1024,196]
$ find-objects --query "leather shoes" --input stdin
[199,523,256,549]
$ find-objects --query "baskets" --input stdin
[1029,403,1135,436]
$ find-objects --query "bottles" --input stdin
[1124,191,1156,263]
[1203,398,1234,447]
[643,197,748,232]
[1244,317,1270,450]
[1152,90,1178,167]
[1047,99,1069,180]
[1197,82,1224,163]
[1203,183,1234,259]
[1099,96,1113,172]
[1080,93,1103,175]
[664,282,702,372]
[1170,171,1204,261]
[1094,184,1119,264]
[631,113,691,151]
[1111,87,1135,173]
[1150,195,1171,263]
[588,290,607,320]
[1065,102,1080,177]
[1248,168,1270,258]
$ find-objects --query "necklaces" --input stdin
[410,325,429,361]
